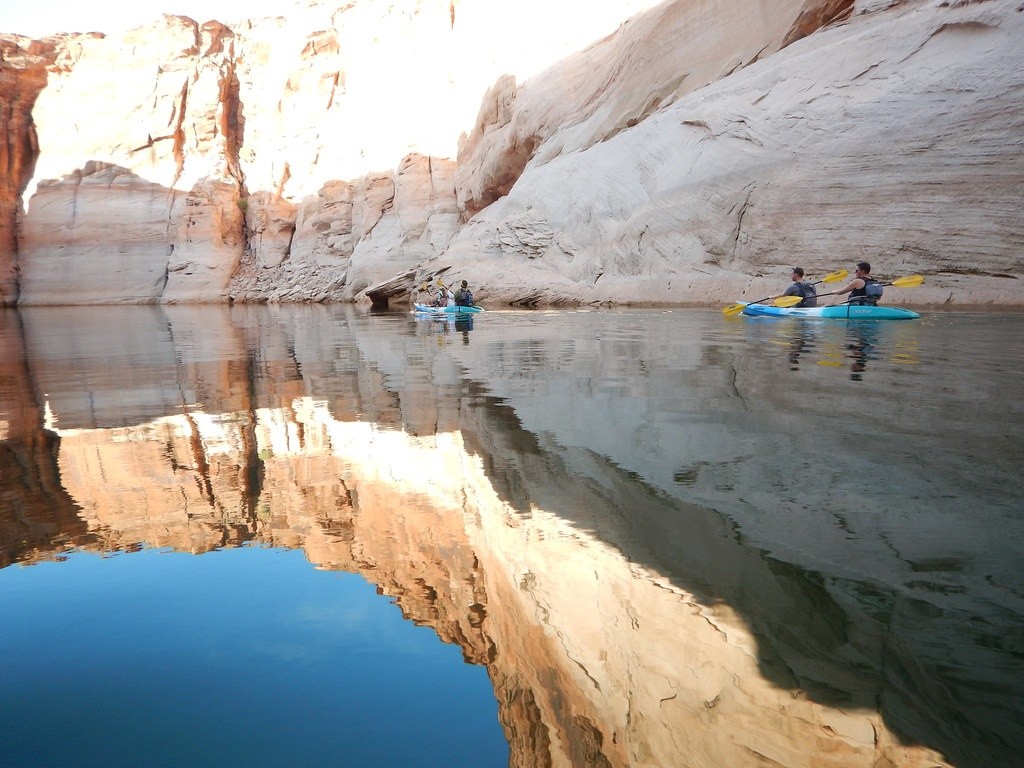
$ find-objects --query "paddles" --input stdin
[722,268,849,317]
[437,280,454,294]
[773,273,924,308]
[422,283,432,297]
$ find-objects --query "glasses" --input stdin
[794,267,798,272]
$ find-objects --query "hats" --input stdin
[461,280,467,288]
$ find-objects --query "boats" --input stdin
[736,295,922,320]
[411,302,446,316]
[443,303,484,315]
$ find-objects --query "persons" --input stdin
[428,280,474,307]
[832,262,883,306]
[768,267,818,308]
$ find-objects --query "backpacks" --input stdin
[465,291,474,306]
